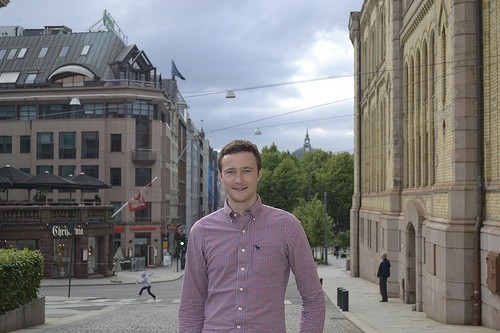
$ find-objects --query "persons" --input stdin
[335,244,340,259]
[136,271,157,301]
[178,139,325,333]
[377,252,391,302]
[95,195,101,205]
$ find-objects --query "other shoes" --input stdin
[380,299,388,302]
[154,296,157,301]
[136,295,141,298]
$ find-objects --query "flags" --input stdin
[128,192,147,212]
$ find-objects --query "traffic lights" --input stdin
[180,236,185,247]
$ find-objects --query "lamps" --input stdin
[69,97,82,105]
[226,91,236,98]
[254,128,262,135]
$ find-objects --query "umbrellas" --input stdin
[0,164,112,206]
